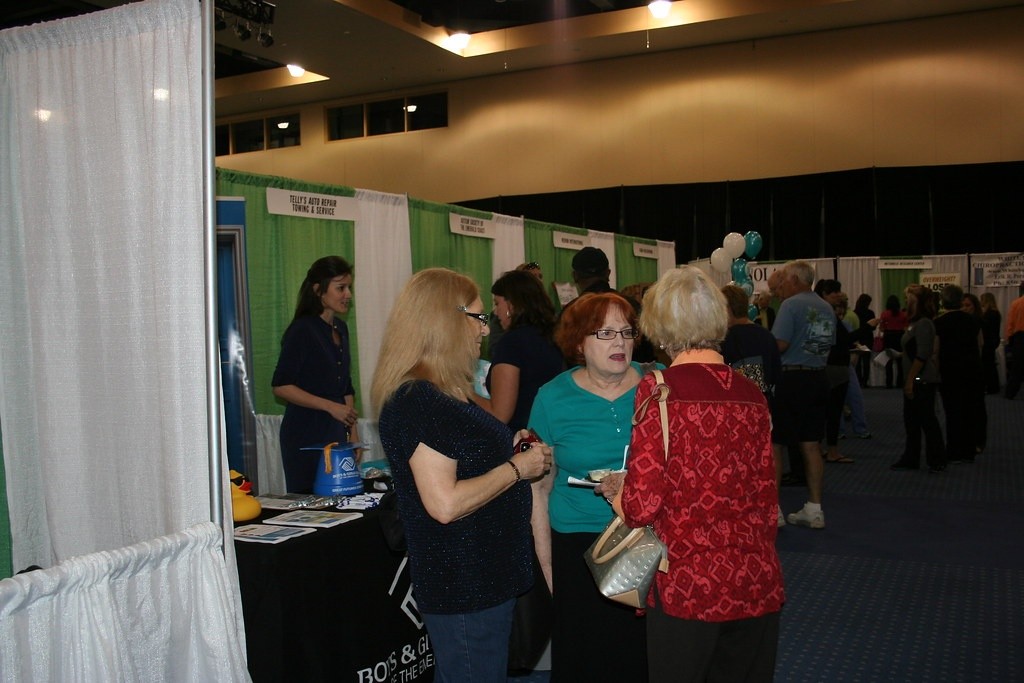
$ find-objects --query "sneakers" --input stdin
[787,502,824,528]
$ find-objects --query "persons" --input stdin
[372,268,552,683]
[558,247,655,364]
[525,295,667,683]
[595,267,788,683]
[722,263,1024,527]
[470,265,566,435]
[271,256,361,493]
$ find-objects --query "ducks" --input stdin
[230,470,261,521]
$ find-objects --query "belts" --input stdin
[781,364,824,371]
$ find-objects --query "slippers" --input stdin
[827,456,856,464]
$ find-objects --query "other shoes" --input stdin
[965,449,983,463]
[838,433,847,440]
[854,431,870,439]
[891,462,919,471]
[928,466,948,473]
[781,472,810,487]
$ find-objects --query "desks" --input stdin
[234,476,439,683]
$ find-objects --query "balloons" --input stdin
[712,231,762,319]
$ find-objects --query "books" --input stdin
[234,494,362,543]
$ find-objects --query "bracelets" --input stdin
[508,460,521,481]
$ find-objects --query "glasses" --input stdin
[522,261,540,272]
[467,312,490,325]
[587,329,639,340]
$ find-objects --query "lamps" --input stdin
[403,99,417,113]
[277,119,289,128]
[215,8,273,48]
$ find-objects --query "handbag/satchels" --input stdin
[583,513,671,607]
